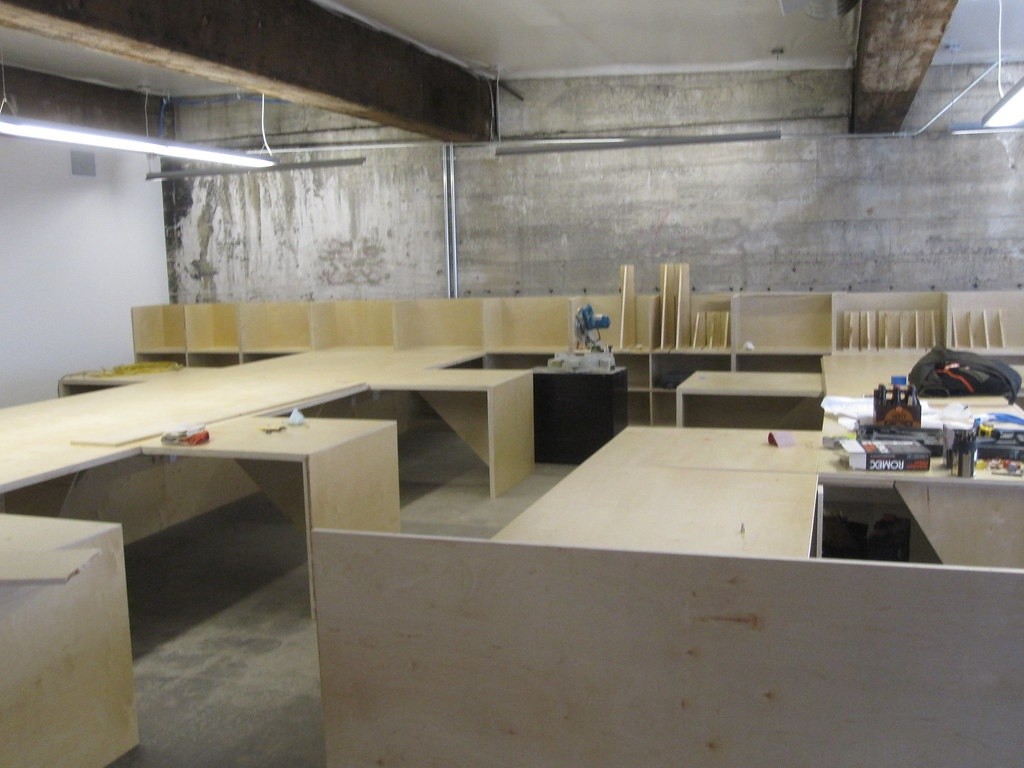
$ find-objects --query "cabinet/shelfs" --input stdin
[132,261,1024,353]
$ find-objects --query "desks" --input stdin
[0,353,1023,768]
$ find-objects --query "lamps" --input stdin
[944,43,1024,134]
[980,0,1024,130]
[0,55,280,170]
